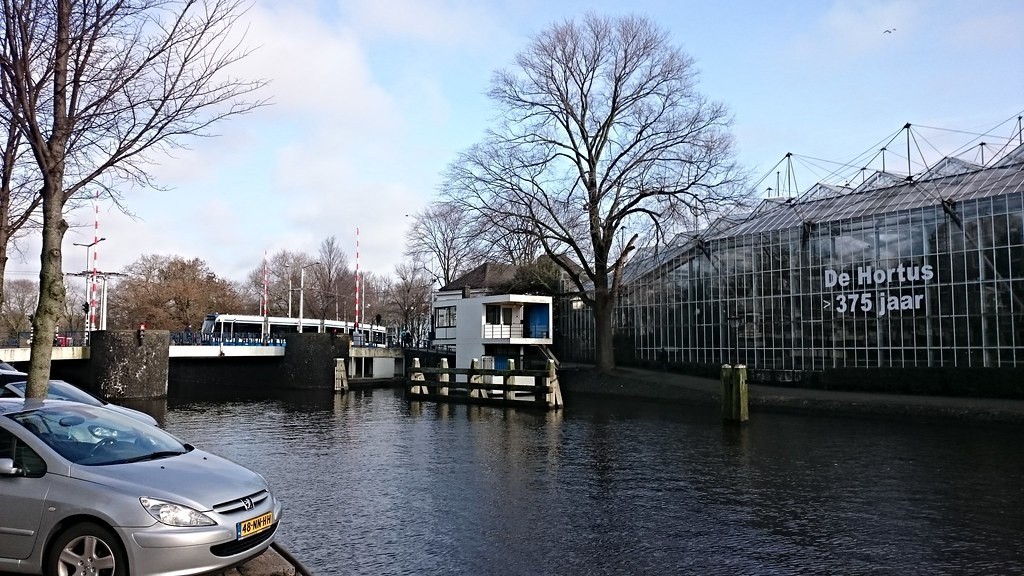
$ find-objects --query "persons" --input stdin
[392,327,411,348]
[184,324,193,344]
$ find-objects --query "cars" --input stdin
[0,359,284,576]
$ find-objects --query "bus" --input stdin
[199,311,389,349]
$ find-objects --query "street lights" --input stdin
[72,237,107,347]
[285,264,292,318]
[295,260,322,333]
